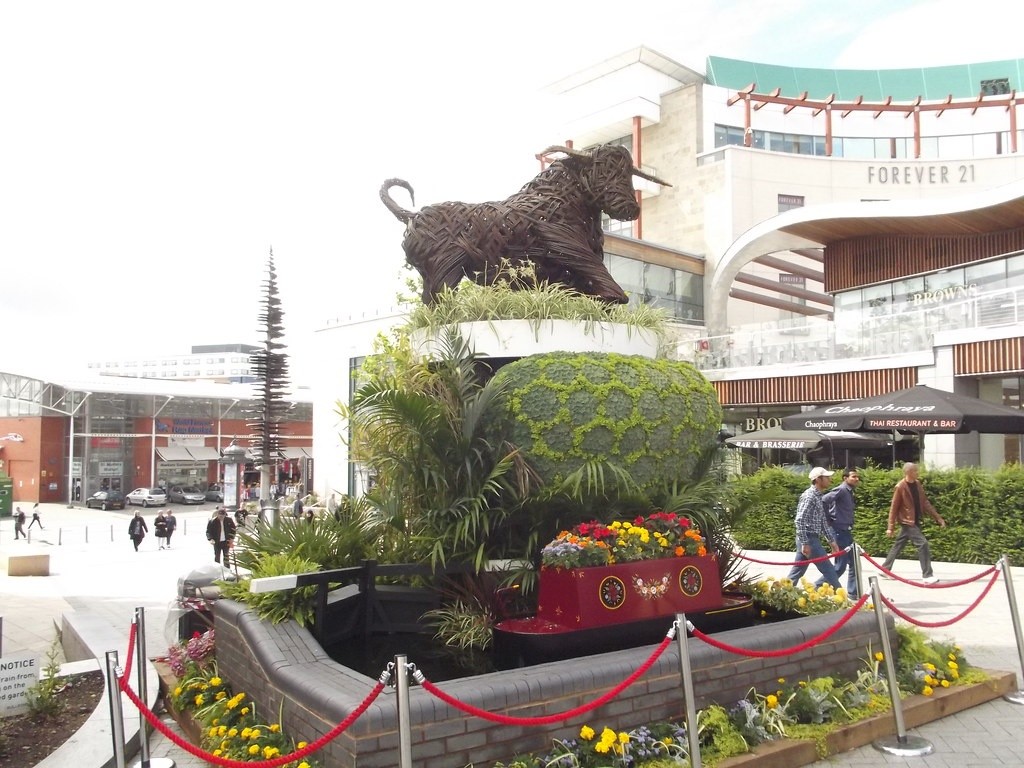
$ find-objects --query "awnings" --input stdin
[156,447,313,462]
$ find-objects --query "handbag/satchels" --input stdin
[299,506,303,514]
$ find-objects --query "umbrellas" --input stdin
[725,383,1024,471]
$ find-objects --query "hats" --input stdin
[809,467,835,482]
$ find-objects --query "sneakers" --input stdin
[923,577,939,584]
[848,595,858,600]
[814,583,819,592]
[878,570,893,580]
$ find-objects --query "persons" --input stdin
[13,507,26,540]
[193,477,207,492]
[27,503,44,529]
[876,463,946,585]
[786,466,842,589]
[128,510,148,552]
[812,469,859,599]
[153,479,349,569]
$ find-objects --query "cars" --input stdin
[203,482,224,503]
[86,491,125,511]
[168,485,207,506]
[126,487,168,508]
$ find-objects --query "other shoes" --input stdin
[14,538,19,540]
[158,543,170,550]
[135,547,138,551]
[24,534,26,538]
[41,527,44,529]
[28,528,31,529]
